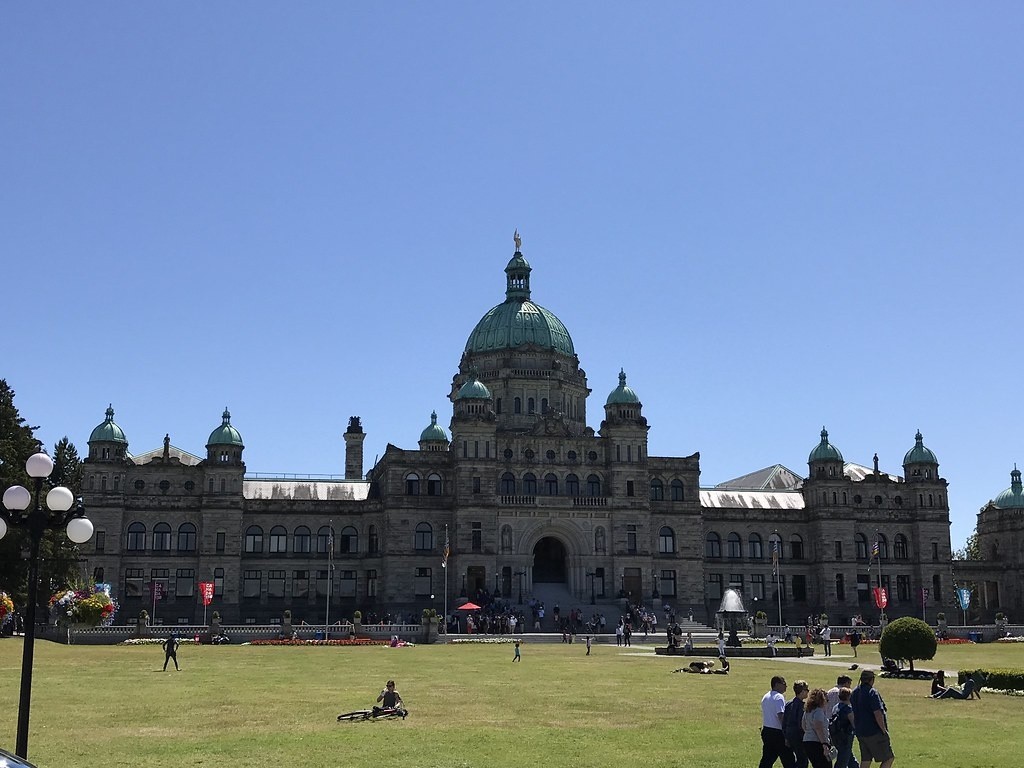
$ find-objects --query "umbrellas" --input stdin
[456,603,481,610]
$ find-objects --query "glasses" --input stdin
[781,683,786,685]
[825,699,829,702]
[805,688,809,692]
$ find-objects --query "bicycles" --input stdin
[337,705,409,721]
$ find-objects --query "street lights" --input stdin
[494,572,502,598]
[618,574,626,598]
[0,452,95,761]
[652,574,659,598]
[461,572,467,597]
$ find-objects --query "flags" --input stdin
[773,542,777,574]
[867,541,879,572]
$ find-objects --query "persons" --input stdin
[290,630,299,639]
[759,657,897,768]
[220,630,226,637]
[512,644,520,662]
[0,610,23,636]
[552,604,606,643]
[936,629,949,641]
[747,615,866,658]
[582,636,594,655]
[377,680,401,708]
[515,234,521,252]
[163,635,181,671]
[663,603,730,674]
[467,600,525,633]
[391,637,397,647]
[530,598,545,629]
[279,632,285,640]
[616,613,632,647]
[625,601,657,634]
[925,670,981,700]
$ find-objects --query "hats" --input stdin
[861,669,876,678]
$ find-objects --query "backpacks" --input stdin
[828,705,849,746]
